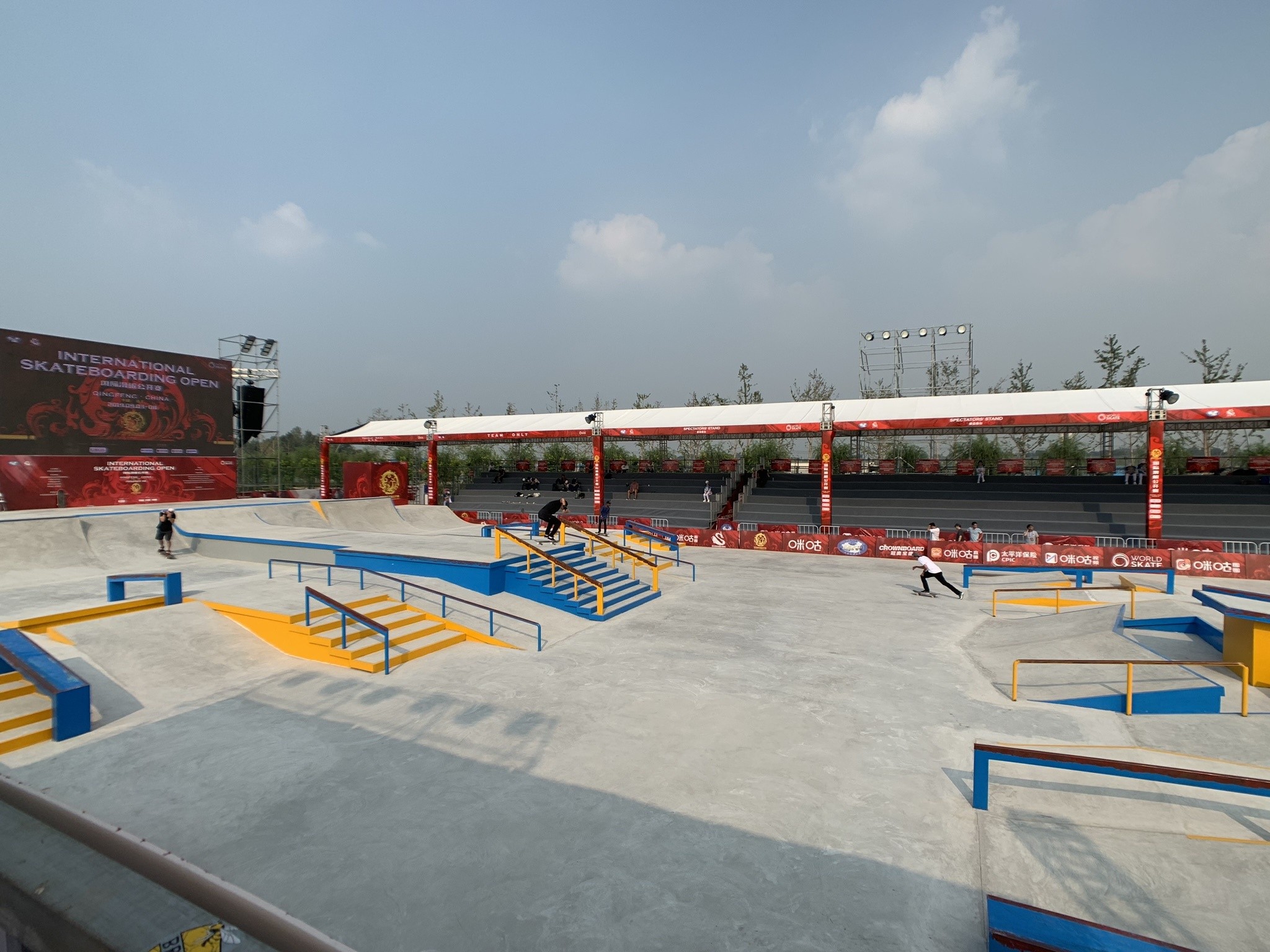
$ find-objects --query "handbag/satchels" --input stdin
[533,492,541,497]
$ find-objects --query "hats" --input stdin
[565,480,568,483]
[705,480,709,483]
[912,552,922,556]
[523,478,525,481]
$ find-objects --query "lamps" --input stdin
[900,329,909,339]
[882,331,891,339]
[1159,389,1179,404]
[938,327,947,336]
[260,338,274,356]
[865,332,874,341]
[424,421,432,429]
[242,336,256,353]
[956,325,966,334]
[585,413,596,424]
[919,328,928,337]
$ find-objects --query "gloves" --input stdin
[563,505,567,511]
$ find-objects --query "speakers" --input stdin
[237,386,265,434]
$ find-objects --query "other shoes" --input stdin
[1139,482,1142,485]
[633,498,636,500]
[548,537,555,541]
[1125,482,1128,485]
[626,498,630,500]
[920,590,930,594]
[708,500,709,502]
[958,592,964,600]
[1134,482,1137,484]
[703,500,706,502]
[544,535,549,537]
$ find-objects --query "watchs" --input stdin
[600,515,602,517]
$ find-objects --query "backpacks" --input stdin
[552,483,558,491]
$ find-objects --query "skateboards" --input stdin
[594,532,609,536]
[535,540,558,545]
[912,590,940,598]
[158,549,177,560]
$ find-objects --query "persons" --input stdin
[538,498,569,541]
[927,523,940,540]
[975,460,986,483]
[912,552,964,600]
[621,461,629,474]
[595,501,612,536]
[491,466,505,484]
[553,474,580,492]
[966,522,984,542]
[521,476,540,490]
[625,480,640,500]
[1137,462,1149,484]
[1124,464,1137,484]
[155,507,175,553]
[756,465,769,487]
[954,523,965,541]
[702,480,713,502]
[1024,525,1039,544]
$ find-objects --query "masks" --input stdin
[607,501,611,504]
